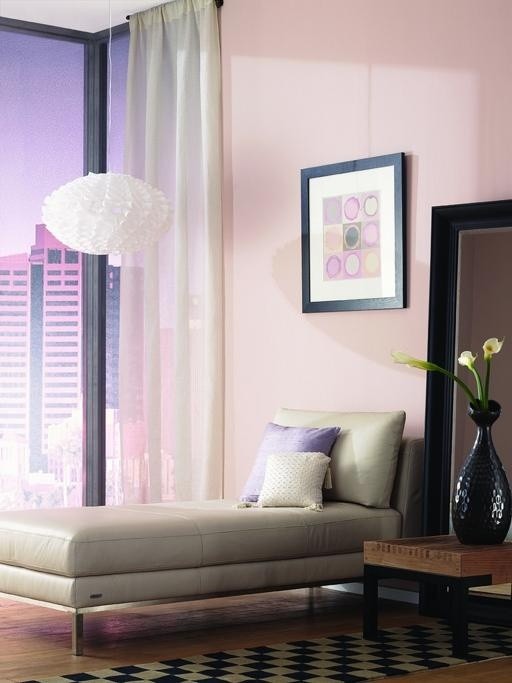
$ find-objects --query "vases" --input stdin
[451,399,512,544]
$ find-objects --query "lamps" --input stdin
[40,2,171,257]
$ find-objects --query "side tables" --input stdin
[358,534,512,659]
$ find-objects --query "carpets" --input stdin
[17,617,511,683]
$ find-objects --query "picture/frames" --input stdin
[300,150,409,312]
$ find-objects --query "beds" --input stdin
[0,439,423,657]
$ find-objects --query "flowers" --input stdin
[390,337,506,411]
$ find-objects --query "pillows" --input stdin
[238,408,407,513]
